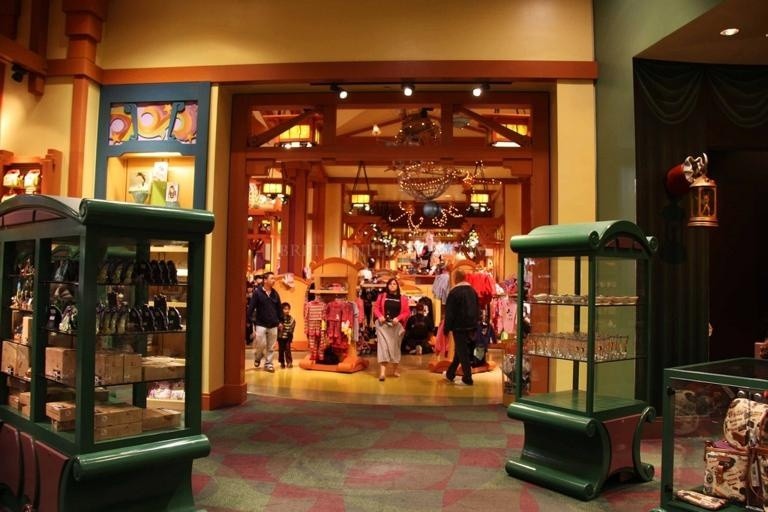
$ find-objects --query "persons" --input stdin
[276,302,296,367]
[404,303,436,356]
[441,267,481,385]
[372,278,410,382]
[246,271,281,373]
[249,275,267,358]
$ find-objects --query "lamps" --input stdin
[251,82,532,212]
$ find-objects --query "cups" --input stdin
[527,331,629,361]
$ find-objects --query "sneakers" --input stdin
[264,366,274,373]
[281,362,293,368]
[254,360,259,367]
[443,371,467,385]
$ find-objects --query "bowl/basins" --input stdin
[532,294,638,306]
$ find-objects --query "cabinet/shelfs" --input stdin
[504,220,664,500]
[652,351,768,512]
[4,199,216,511]
[0,147,67,194]
[298,257,497,376]
